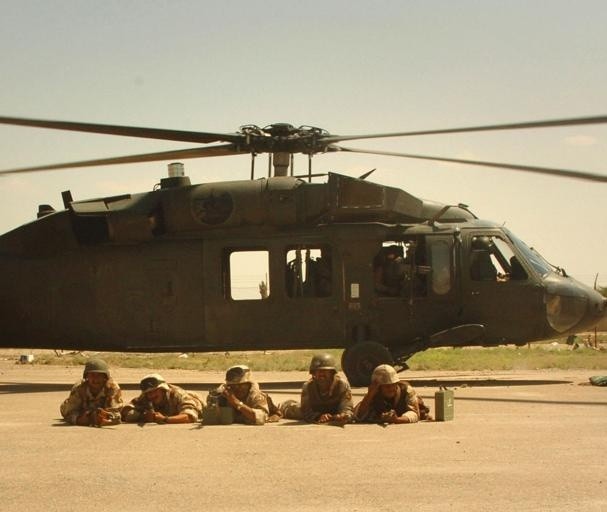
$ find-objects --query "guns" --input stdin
[372,411,397,423]
[327,414,352,428]
[214,384,232,406]
[86,400,114,428]
[143,399,155,421]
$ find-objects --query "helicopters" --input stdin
[0,116,607,387]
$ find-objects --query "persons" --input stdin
[202,364,280,425]
[274,352,353,424]
[57,359,124,426]
[351,363,431,424]
[121,371,205,425]
[371,244,414,296]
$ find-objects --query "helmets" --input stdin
[83,359,111,380]
[140,374,170,393]
[371,364,400,385]
[310,352,338,374]
[226,365,254,387]
